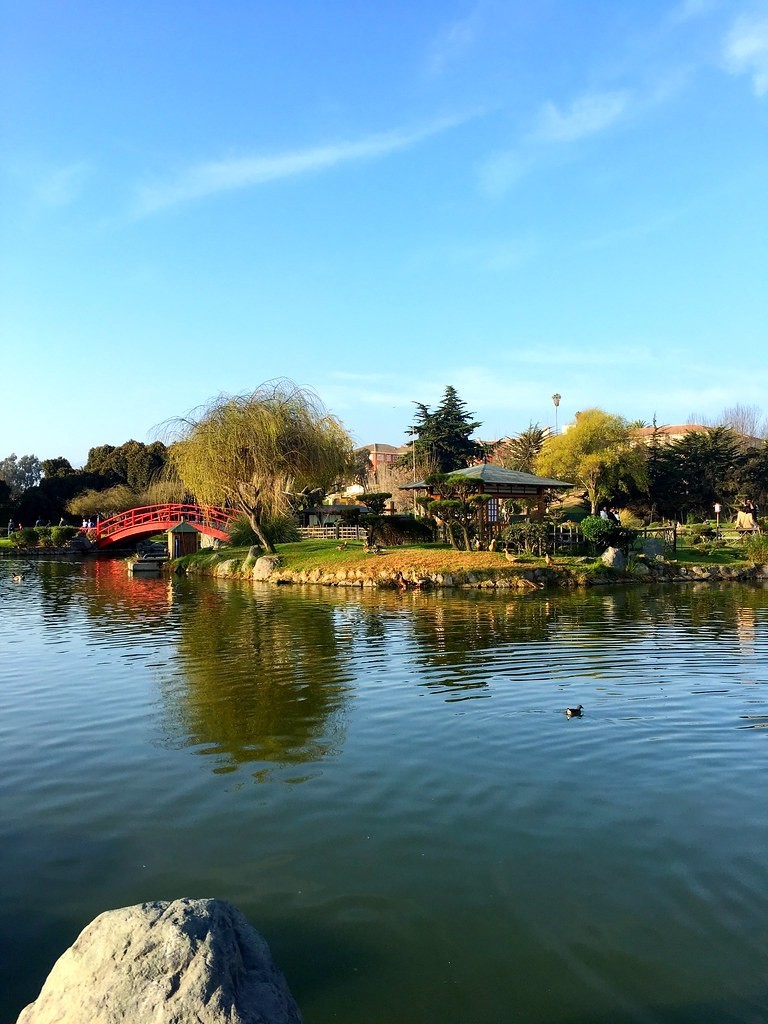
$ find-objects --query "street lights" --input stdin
[392,404,420,521]
[552,392,562,436]
[713,501,722,542]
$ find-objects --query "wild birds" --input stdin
[336,541,348,553]
[565,703,585,719]
[396,571,412,591]
[502,547,521,565]
[11,571,28,582]
[488,538,497,552]
[544,553,554,566]
[361,540,380,556]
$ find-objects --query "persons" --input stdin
[36,516,52,526]
[82,519,94,532]
[19,523,22,530]
[8,519,15,535]
[59,517,68,526]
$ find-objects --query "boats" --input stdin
[126,558,162,572]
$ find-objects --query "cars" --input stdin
[693,532,724,545]
